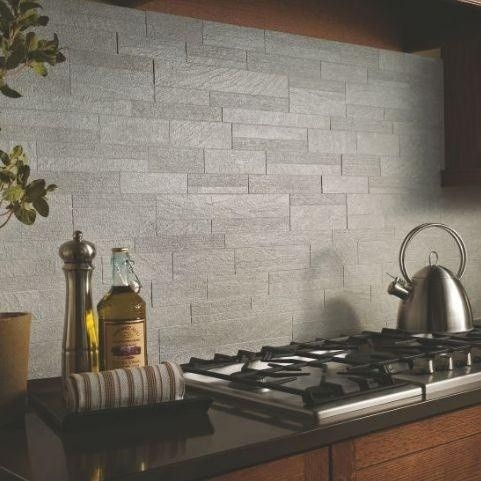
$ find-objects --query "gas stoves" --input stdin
[180,319,481,421]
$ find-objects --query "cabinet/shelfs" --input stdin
[205,403,481,481]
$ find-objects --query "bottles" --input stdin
[97,247,148,372]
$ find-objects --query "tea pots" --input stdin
[388,220,476,337]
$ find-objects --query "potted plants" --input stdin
[1,0,59,408]
